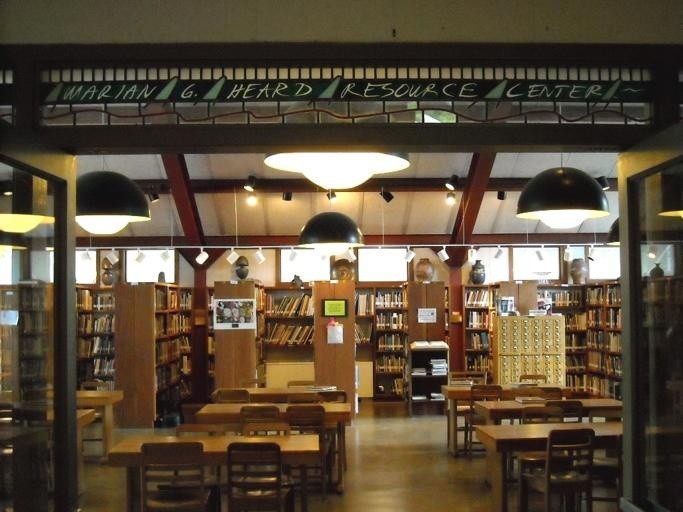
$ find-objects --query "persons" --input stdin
[354,323,373,343]
[273,295,314,317]
[267,324,314,345]
[356,294,375,317]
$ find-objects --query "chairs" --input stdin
[138,441,220,512]
[285,406,325,434]
[448,371,487,447]
[519,375,547,384]
[226,442,309,512]
[314,391,344,403]
[464,385,502,457]
[176,424,225,436]
[243,420,290,437]
[240,405,280,421]
[589,409,623,423]
[518,406,569,474]
[287,381,315,387]
[519,429,595,512]
[80,382,109,391]
[511,387,545,399]
[238,379,265,387]
[544,400,583,462]
[216,389,251,403]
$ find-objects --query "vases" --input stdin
[414,259,435,281]
[330,260,354,280]
[570,258,587,284]
[650,264,664,278]
[471,260,486,284]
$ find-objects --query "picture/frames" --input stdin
[323,298,348,317]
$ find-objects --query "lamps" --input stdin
[106,252,120,265]
[564,245,574,262]
[327,192,336,203]
[497,190,508,201]
[226,252,240,264]
[379,192,394,203]
[243,175,258,192]
[296,190,365,257]
[346,247,357,263]
[589,245,597,261]
[246,193,258,206]
[517,153,610,228]
[447,194,456,205]
[437,246,449,262]
[536,246,544,261]
[282,191,293,201]
[290,247,298,261]
[76,154,150,234]
[0,190,55,233]
[195,252,209,265]
[656,176,683,217]
[255,252,266,264]
[495,246,503,260]
[445,174,459,191]
[647,244,658,260]
[136,252,145,263]
[404,246,417,262]
[606,217,621,245]
[596,176,609,190]
[263,147,412,190]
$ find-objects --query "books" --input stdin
[586,284,622,304]
[468,311,489,327]
[588,331,622,351]
[155,315,165,338]
[79,315,94,333]
[76,288,93,313]
[94,314,115,332]
[376,313,407,332]
[154,341,166,365]
[567,373,584,391]
[375,287,405,310]
[309,385,336,389]
[256,314,265,339]
[94,290,114,312]
[180,291,192,309]
[93,359,114,378]
[165,339,179,363]
[256,365,265,388]
[255,285,266,312]
[179,337,189,352]
[564,330,586,353]
[155,290,168,311]
[79,338,100,357]
[375,376,403,395]
[377,334,404,354]
[413,391,448,402]
[375,356,403,370]
[168,314,177,337]
[412,357,448,377]
[586,378,623,400]
[588,353,620,374]
[256,342,262,362]
[566,353,586,368]
[179,313,190,332]
[105,336,114,357]
[158,365,181,389]
[182,357,192,373]
[169,291,177,311]
[467,351,491,371]
[467,290,493,306]
[587,308,620,329]
[517,395,545,403]
[562,311,586,330]
[410,341,448,350]
[470,331,489,349]
[537,287,584,306]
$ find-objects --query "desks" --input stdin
[211,386,337,402]
[77,408,95,496]
[474,399,622,425]
[477,421,623,512]
[78,391,124,451]
[442,385,570,457]
[108,434,319,512]
[197,402,353,494]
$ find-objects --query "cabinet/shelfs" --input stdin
[355,281,407,401]
[642,277,683,412]
[180,286,213,402]
[463,284,489,378]
[264,287,313,346]
[492,315,565,387]
[489,280,537,377]
[537,284,585,391]
[406,282,449,415]
[1,286,19,392]
[114,283,180,428]
[16,284,94,389]
[585,281,623,401]
[93,286,115,390]
[214,281,266,388]
[313,281,354,420]
